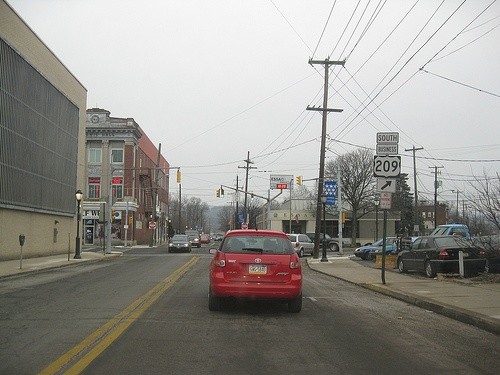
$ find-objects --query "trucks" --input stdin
[429,224,489,243]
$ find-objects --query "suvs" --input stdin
[304,232,340,253]
[184,230,202,248]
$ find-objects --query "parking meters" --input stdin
[149,222,156,229]
[19,233,26,270]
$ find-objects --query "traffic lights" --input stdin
[296,176,302,186]
[342,213,346,224]
[112,208,115,220]
[129,216,133,225]
[216,189,221,198]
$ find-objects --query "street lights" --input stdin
[150,173,170,246]
[73,189,84,259]
[434,188,455,230]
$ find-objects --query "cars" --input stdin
[396,235,487,278]
[207,228,304,313]
[200,233,224,244]
[353,236,420,262]
[284,233,314,257]
[168,234,192,253]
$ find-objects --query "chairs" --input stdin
[264,239,277,249]
[229,239,245,249]
